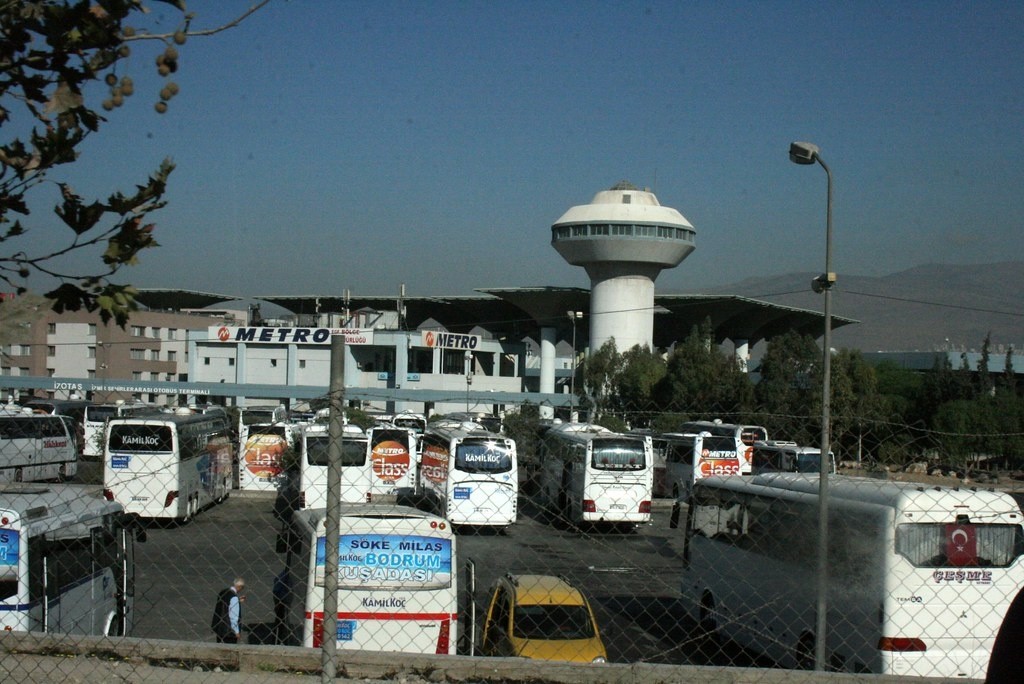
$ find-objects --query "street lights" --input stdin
[787,141,837,676]
[464,354,474,419]
[566,310,575,422]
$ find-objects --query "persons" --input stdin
[211,575,247,645]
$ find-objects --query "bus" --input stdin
[533,422,653,528]
[617,417,837,500]
[0,395,525,529]
[0,481,148,638]
[669,471,1024,680]
[271,503,477,658]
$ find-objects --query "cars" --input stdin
[478,572,610,664]
